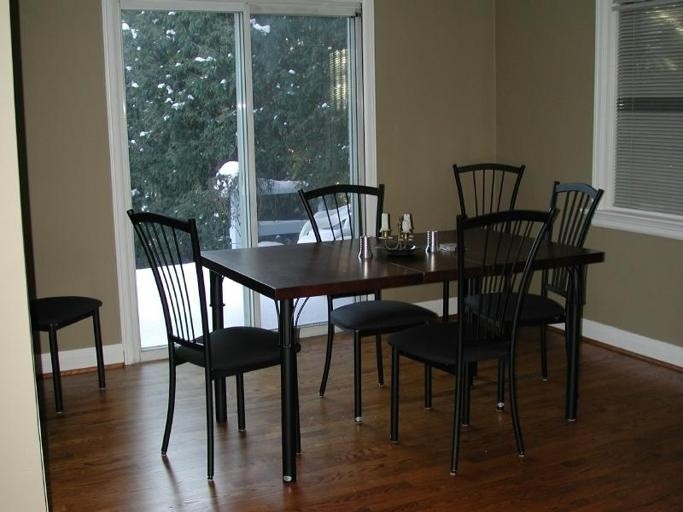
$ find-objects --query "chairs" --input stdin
[259,204,353,246]
[126,206,305,484]
[451,160,528,388]
[383,205,562,480]
[297,181,440,423]
[460,178,605,410]
[26,295,107,419]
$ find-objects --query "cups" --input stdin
[359,235,373,259]
[426,229,441,255]
[427,255,439,272]
[359,260,372,278]
